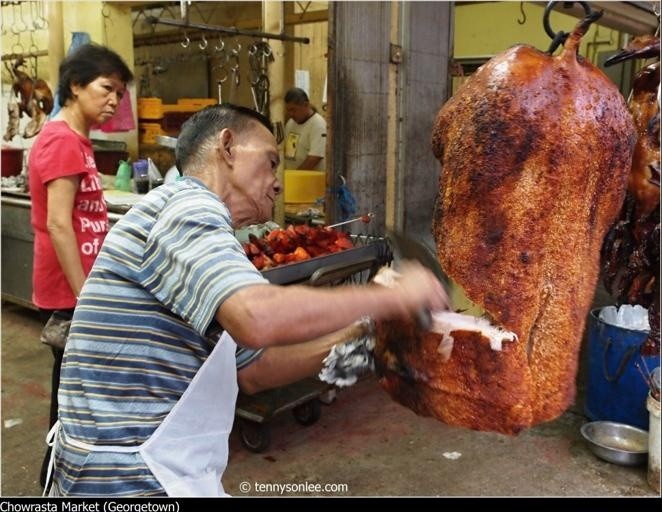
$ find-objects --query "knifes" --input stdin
[382,227,454,333]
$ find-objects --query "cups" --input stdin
[133,160,150,195]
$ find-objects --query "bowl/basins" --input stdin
[580,420,649,466]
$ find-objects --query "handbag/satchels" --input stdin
[40,311,72,350]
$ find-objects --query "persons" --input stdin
[40,101,456,499]
[25,42,134,494]
[279,87,327,174]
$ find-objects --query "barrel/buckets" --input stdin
[584,305,660,427]
[644,366,661,493]
